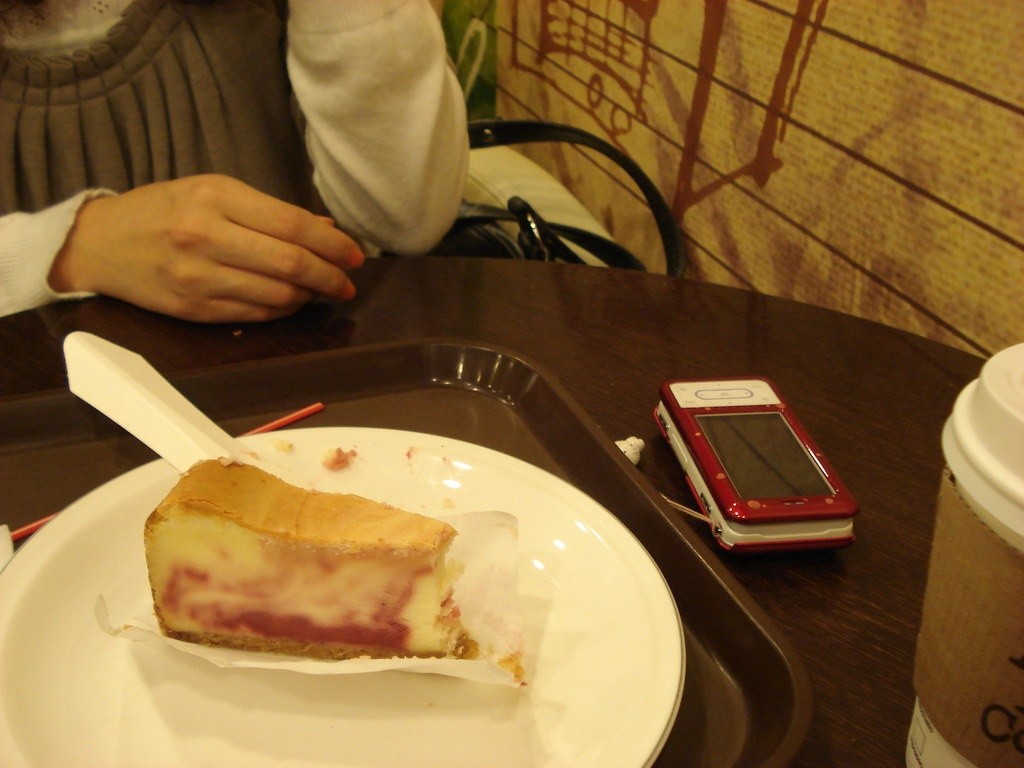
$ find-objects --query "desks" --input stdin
[1,250,993,768]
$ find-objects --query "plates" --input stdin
[0,426,685,768]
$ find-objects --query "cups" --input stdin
[906,341,1024,768]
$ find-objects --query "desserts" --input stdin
[144,458,471,660]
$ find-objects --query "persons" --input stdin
[0,0,469,324]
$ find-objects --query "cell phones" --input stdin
[654,375,860,552]
